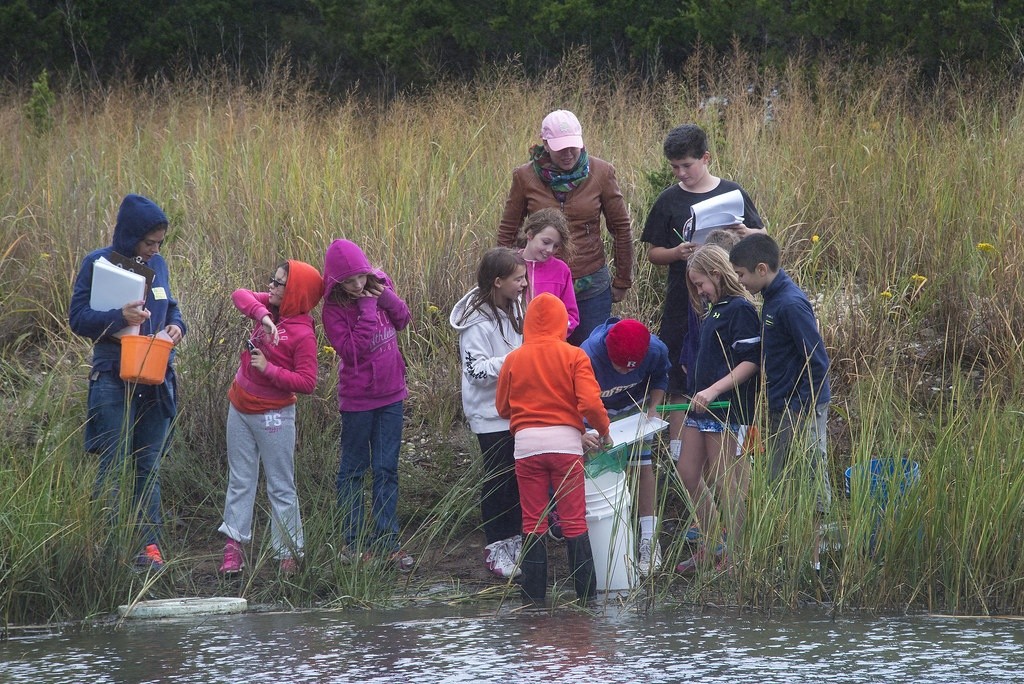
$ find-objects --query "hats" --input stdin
[541,110,584,151]
[606,319,650,367]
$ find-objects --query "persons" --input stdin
[676,228,762,584]
[729,234,830,570]
[497,109,633,344]
[449,248,528,579]
[70,193,186,576]
[219,260,323,576]
[578,317,671,576]
[496,293,615,599]
[641,124,767,485]
[322,240,415,573]
[516,207,579,542]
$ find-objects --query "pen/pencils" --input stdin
[673,228,693,252]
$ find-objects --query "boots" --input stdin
[566,530,597,598]
[521,532,548,601]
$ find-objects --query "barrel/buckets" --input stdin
[120,335,174,385]
[845,458,921,558]
[584,472,640,590]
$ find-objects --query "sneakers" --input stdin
[281,560,299,575]
[676,544,738,582]
[219,543,246,575]
[637,539,662,577]
[505,535,523,563]
[485,540,522,579]
[389,551,417,572]
[135,544,168,573]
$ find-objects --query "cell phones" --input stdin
[247,340,257,356]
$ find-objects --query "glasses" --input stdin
[269,277,286,288]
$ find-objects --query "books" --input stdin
[690,190,745,243]
[90,253,156,339]
